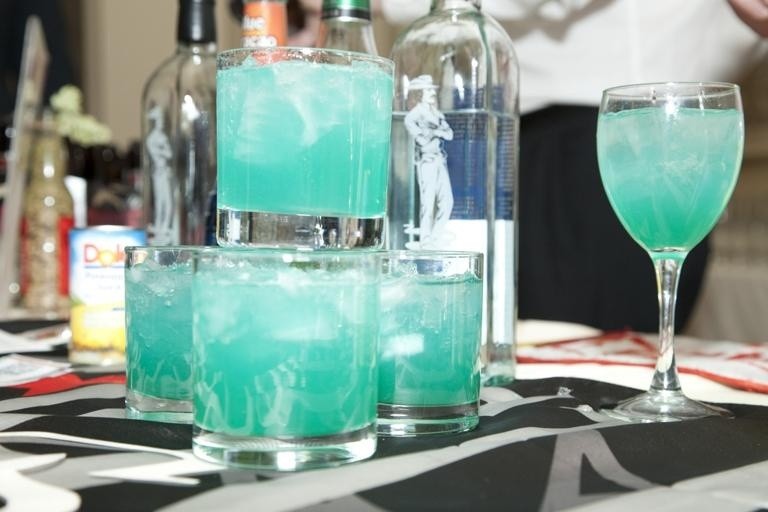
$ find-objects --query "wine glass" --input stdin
[595,78,746,424]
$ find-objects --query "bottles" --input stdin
[389,1,522,388]
[235,0,379,55]
[16,107,76,311]
[139,0,222,247]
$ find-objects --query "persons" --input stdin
[403,82,455,248]
[274,1,768,335]
[144,104,180,247]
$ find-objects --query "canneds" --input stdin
[68,225,147,366]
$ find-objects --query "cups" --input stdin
[373,247,486,441]
[122,244,204,426]
[184,244,378,473]
[212,45,398,254]
